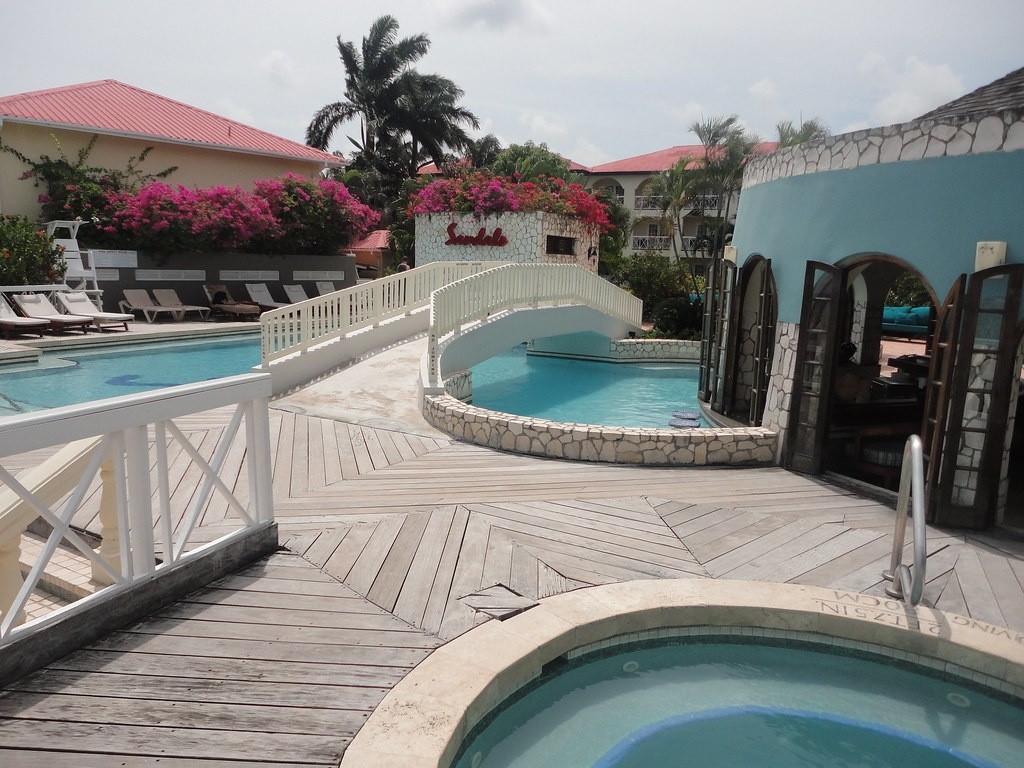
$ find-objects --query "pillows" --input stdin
[895,313,919,325]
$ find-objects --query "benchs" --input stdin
[881,306,930,341]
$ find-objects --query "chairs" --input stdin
[153,289,211,322]
[13,293,94,335]
[203,284,261,321]
[283,284,333,310]
[54,292,135,333]
[316,281,363,306]
[246,283,293,308]
[0,293,50,340]
[118,290,183,323]
